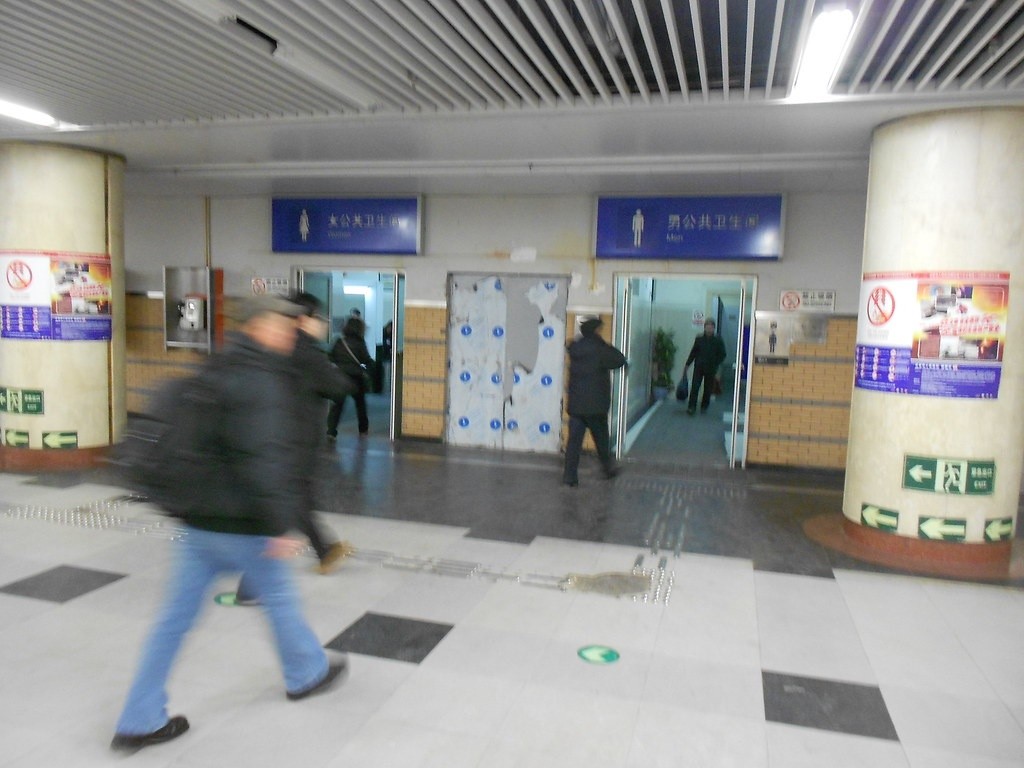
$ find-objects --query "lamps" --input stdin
[792,2,853,103]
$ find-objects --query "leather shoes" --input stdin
[111,715,191,753]
[284,664,348,701]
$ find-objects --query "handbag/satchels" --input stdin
[362,368,374,393]
[676,369,688,401]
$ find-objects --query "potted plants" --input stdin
[649,325,676,400]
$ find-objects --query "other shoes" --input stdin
[686,408,693,416]
[565,479,578,488]
[606,466,624,480]
[233,593,264,607]
[700,409,706,414]
[318,543,351,576]
[328,433,336,439]
[360,432,368,438]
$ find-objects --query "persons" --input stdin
[384,320,392,354]
[109,296,346,750]
[683,317,727,415]
[562,318,627,486]
[326,316,379,442]
[233,290,351,605]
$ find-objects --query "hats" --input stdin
[245,295,308,317]
